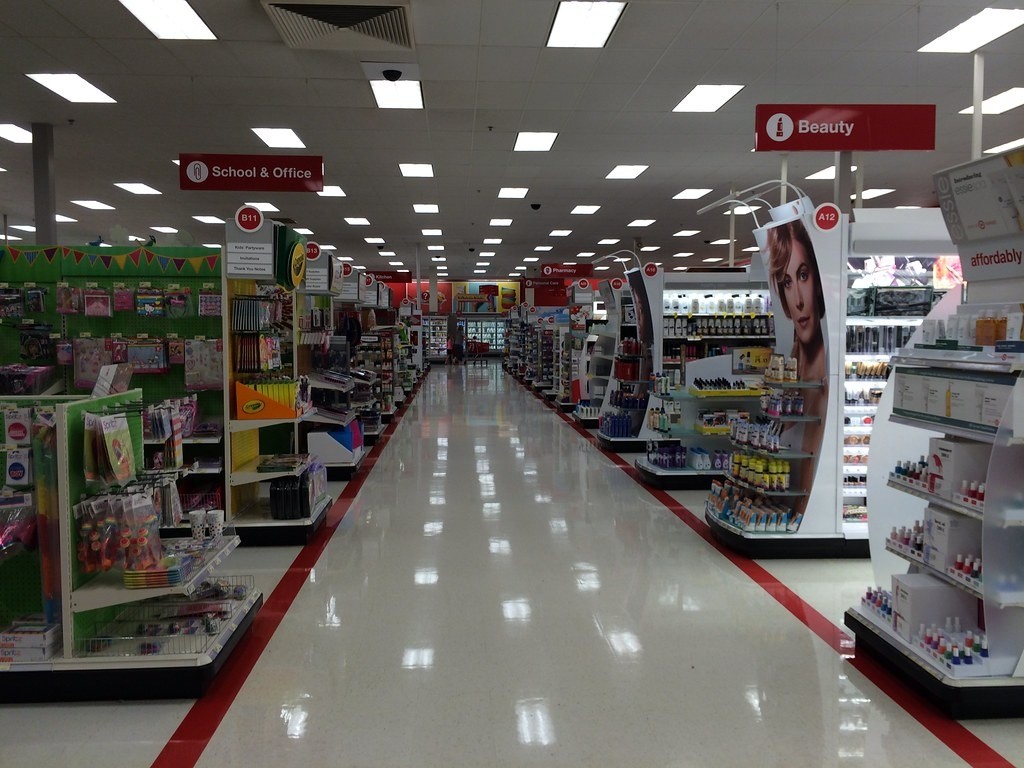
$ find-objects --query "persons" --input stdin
[472,336,478,342]
[769,219,828,514]
[445,335,455,365]
[452,325,468,365]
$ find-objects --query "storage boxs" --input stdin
[307,421,365,462]
[890,573,979,644]
[927,437,992,499]
[592,386,604,394]
[302,403,309,413]
[235,380,303,419]
[924,507,981,573]
[308,401,312,410]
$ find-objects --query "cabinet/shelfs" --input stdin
[455,146,1024,718]
[0,204,450,700]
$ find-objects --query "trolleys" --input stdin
[464,338,490,365]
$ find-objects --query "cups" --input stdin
[189,510,206,540]
[207,510,224,539]
